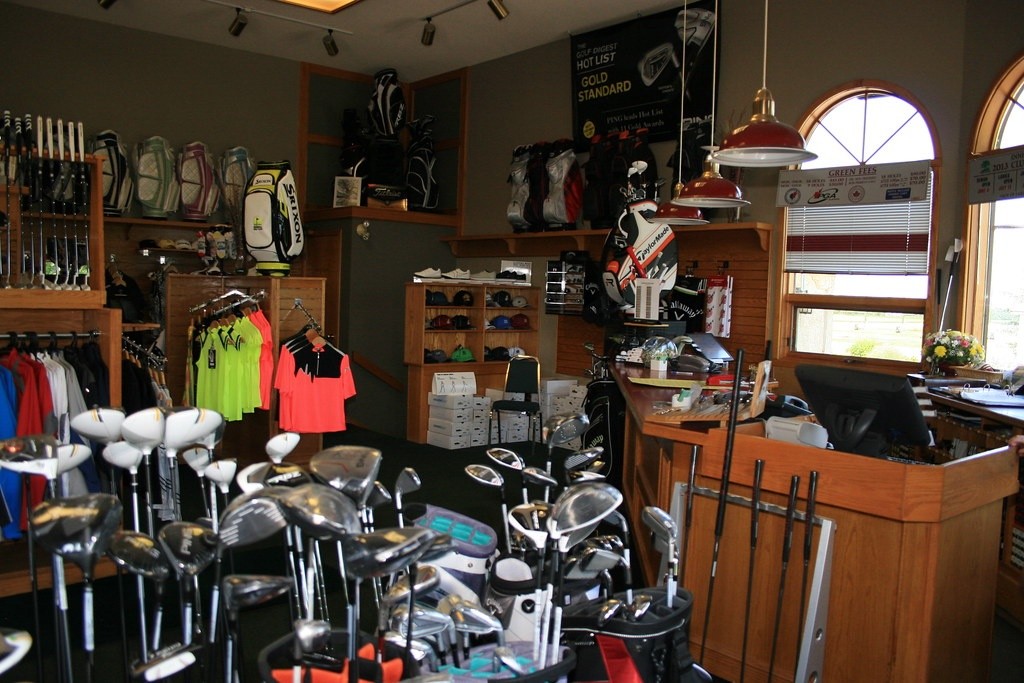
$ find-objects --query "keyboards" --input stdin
[887,455,935,465]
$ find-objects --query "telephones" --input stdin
[758,393,813,420]
[676,353,709,373]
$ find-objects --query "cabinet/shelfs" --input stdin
[908,373,1024,635]
[0,186,231,253]
[604,361,745,587]
[403,283,540,442]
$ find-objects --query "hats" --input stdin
[157,239,177,249]
[426,289,473,306]
[424,344,477,363]
[485,313,530,330]
[425,315,472,330]
[484,345,526,361]
[139,238,161,249]
[175,239,192,250]
[485,291,529,309]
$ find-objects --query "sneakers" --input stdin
[414,268,526,283]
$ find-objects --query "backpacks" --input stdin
[243,161,304,263]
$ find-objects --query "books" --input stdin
[948,383,1024,408]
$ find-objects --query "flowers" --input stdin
[923,329,986,368]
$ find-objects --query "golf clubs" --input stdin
[0,347,817,683]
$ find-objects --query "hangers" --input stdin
[186,294,261,346]
[0,331,94,357]
[121,333,173,409]
[303,317,327,347]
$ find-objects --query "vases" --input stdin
[940,362,965,374]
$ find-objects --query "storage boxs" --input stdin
[427,379,587,450]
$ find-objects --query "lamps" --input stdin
[709,0,816,167]
[323,30,339,56]
[98,0,116,9]
[671,0,751,210]
[487,0,509,20]
[228,9,247,37]
[422,18,435,46]
[647,0,710,224]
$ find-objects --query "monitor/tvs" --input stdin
[795,362,931,459]
[685,332,734,362]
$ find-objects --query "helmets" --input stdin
[600,209,679,307]
